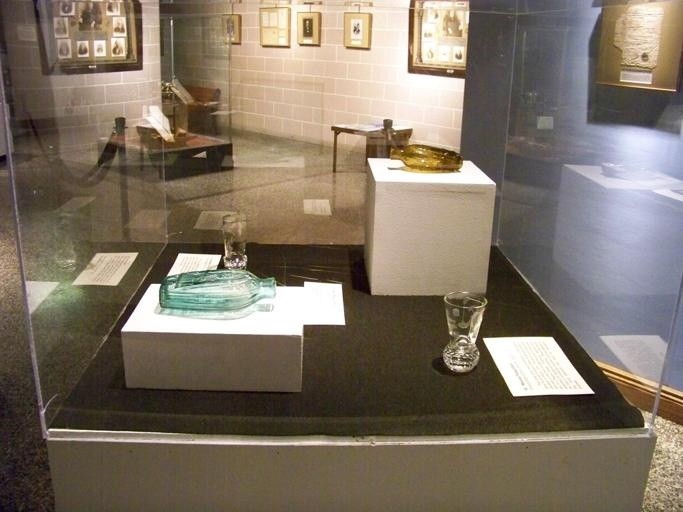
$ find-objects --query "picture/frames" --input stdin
[407,0,469,80]
[32,0,144,77]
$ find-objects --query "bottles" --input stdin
[159,268,277,311]
[390,144,463,174]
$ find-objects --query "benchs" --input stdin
[161,84,221,137]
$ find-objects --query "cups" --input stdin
[222,213,248,270]
[442,290,488,373]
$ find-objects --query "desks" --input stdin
[95,127,234,180]
[330,121,413,173]
